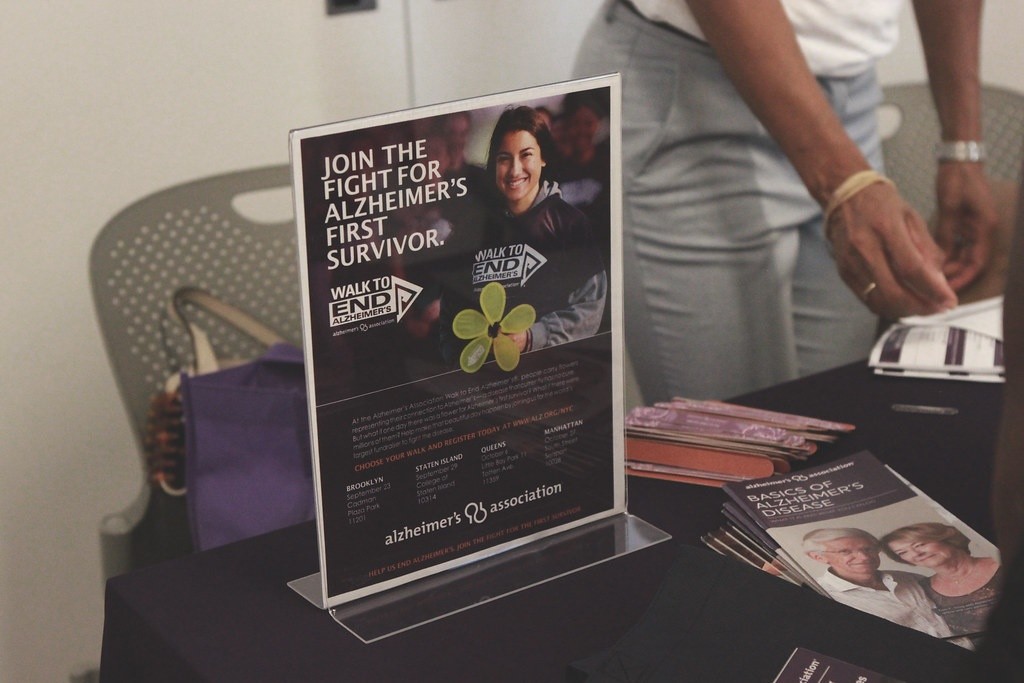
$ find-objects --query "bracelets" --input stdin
[935,139,986,162]
[823,171,896,238]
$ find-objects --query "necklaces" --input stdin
[952,580,961,585]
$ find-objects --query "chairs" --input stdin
[89,165,302,593]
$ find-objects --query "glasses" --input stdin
[816,545,881,555]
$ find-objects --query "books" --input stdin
[699,450,1003,683]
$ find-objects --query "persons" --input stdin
[572,0,993,407]
[802,522,973,653]
[343,93,612,392]
[880,523,1002,648]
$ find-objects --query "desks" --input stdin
[98,347,1006,683]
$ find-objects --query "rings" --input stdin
[863,281,878,295]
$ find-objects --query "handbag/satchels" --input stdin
[173,288,316,556]
